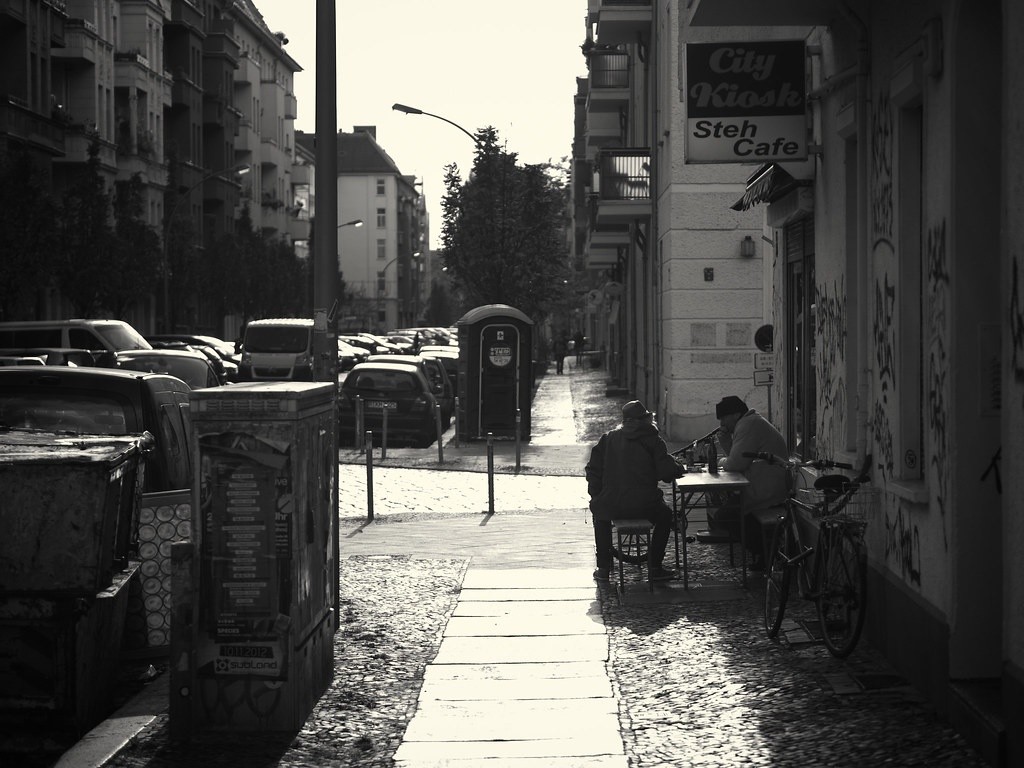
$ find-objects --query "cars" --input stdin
[0,319,241,389]
[0,365,195,493]
[336,362,442,449]
[364,354,454,433]
[567,336,593,352]
[337,327,460,389]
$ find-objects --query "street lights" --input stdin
[306,219,362,318]
[376,250,422,335]
[163,164,250,335]
[392,104,496,305]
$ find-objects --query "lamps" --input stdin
[740,234,756,259]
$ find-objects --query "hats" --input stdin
[622,400,649,419]
[716,396,749,419]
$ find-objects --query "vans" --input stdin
[238,319,317,381]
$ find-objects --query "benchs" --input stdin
[749,505,792,577]
[611,515,653,593]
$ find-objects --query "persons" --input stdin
[716,396,792,572]
[548,328,570,376]
[572,328,588,367]
[584,400,684,582]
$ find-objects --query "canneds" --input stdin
[685,450,694,469]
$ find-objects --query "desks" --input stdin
[672,471,751,594]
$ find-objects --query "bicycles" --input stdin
[742,451,868,657]
[610,425,724,562]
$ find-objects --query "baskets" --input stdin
[799,483,881,521]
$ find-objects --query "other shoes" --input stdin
[593,569,608,580]
[648,565,679,580]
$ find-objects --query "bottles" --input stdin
[708,437,717,473]
[685,450,694,467]
